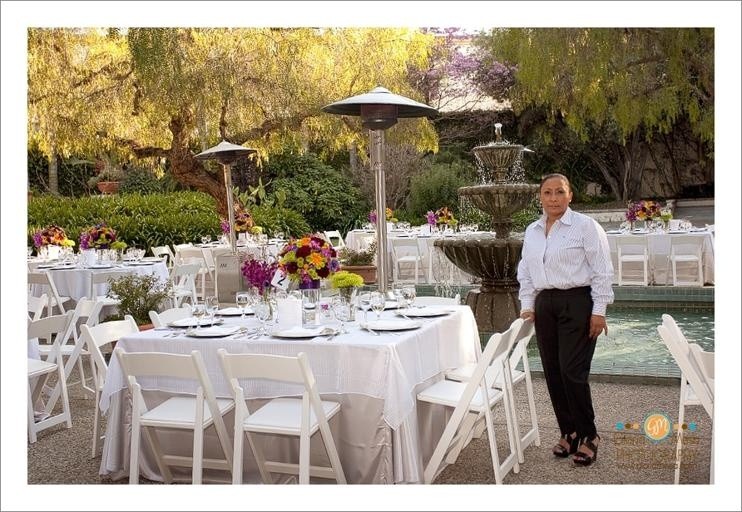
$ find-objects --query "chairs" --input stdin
[28,220,714,485]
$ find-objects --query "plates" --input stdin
[397,234,459,238]
[396,308,448,319]
[37,262,153,270]
[215,307,255,317]
[187,325,240,339]
[606,229,707,234]
[270,328,340,339]
[168,319,220,327]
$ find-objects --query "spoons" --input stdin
[232,327,259,340]
[359,322,378,335]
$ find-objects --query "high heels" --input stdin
[553,433,579,458]
[573,433,600,466]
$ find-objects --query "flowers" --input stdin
[625,197,663,221]
[368,207,399,225]
[425,206,459,225]
[220,205,254,233]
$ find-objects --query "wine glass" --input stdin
[206,295,218,326]
[235,291,249,320]
[255,301,274,334]
[362,221,414,231]
[200,232,285,248]
[459,224,478,235]
[333,286,418,333]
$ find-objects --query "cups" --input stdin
[27,245,146,265]
[670,219,681,231]
[300,289,318,309]
[433,224,452,235]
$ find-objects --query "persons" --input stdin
[517,173,615,465]
